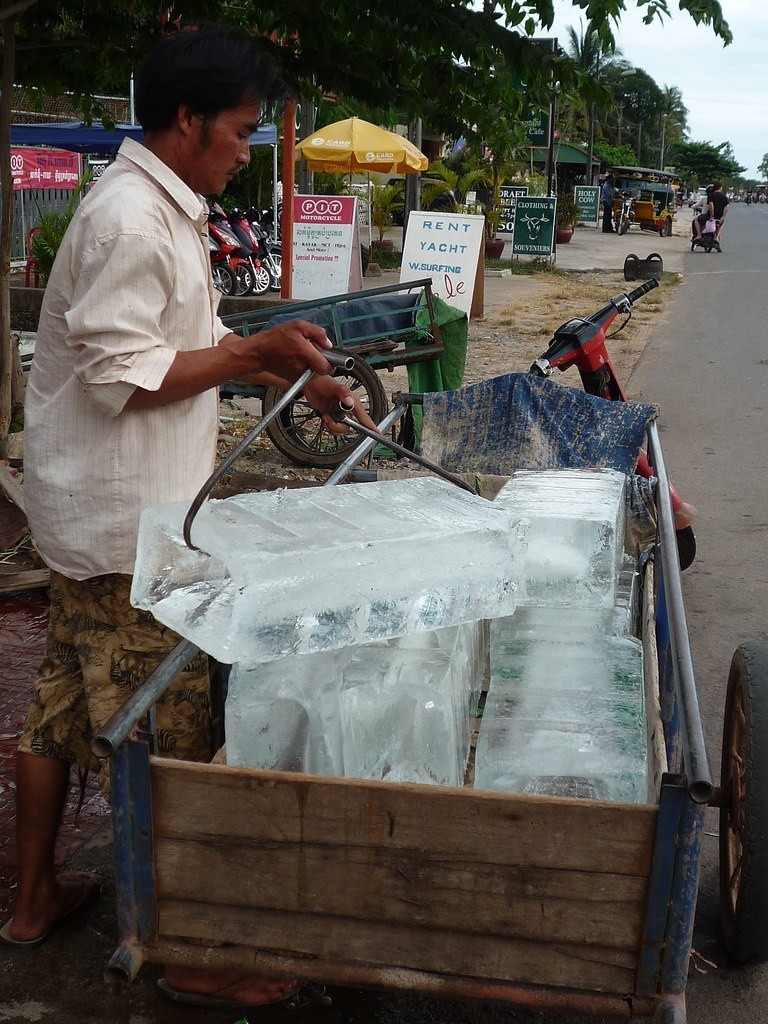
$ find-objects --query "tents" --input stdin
[8,121,279,259]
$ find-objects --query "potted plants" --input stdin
[479,128,527,262]
[366,175,405,255]
[555,185,581,244]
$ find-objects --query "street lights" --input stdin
[585,69,637,186]
[659,114,682,172]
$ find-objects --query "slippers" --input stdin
[155,970,325,1011]
[0,868,103,948]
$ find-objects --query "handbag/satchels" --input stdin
[702,220,716,233]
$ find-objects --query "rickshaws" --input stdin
[21,277,445,467]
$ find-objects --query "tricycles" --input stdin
[90,277,767,1024]
[606,166,679,238]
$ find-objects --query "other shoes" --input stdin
[602,229,616,233]
[694,237,701,240]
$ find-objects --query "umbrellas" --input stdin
[294,116,429,264]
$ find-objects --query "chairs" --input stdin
[24,226,48,288]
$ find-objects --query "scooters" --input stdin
[726,194,768,206]
[207,201,284,298]
[690,206,721,253]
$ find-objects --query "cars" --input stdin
[338,172,376,206]
[686,187,708,208]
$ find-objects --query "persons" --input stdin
[691,182,768,252]
[1,26,378,1010]
[600,174,615,232]
